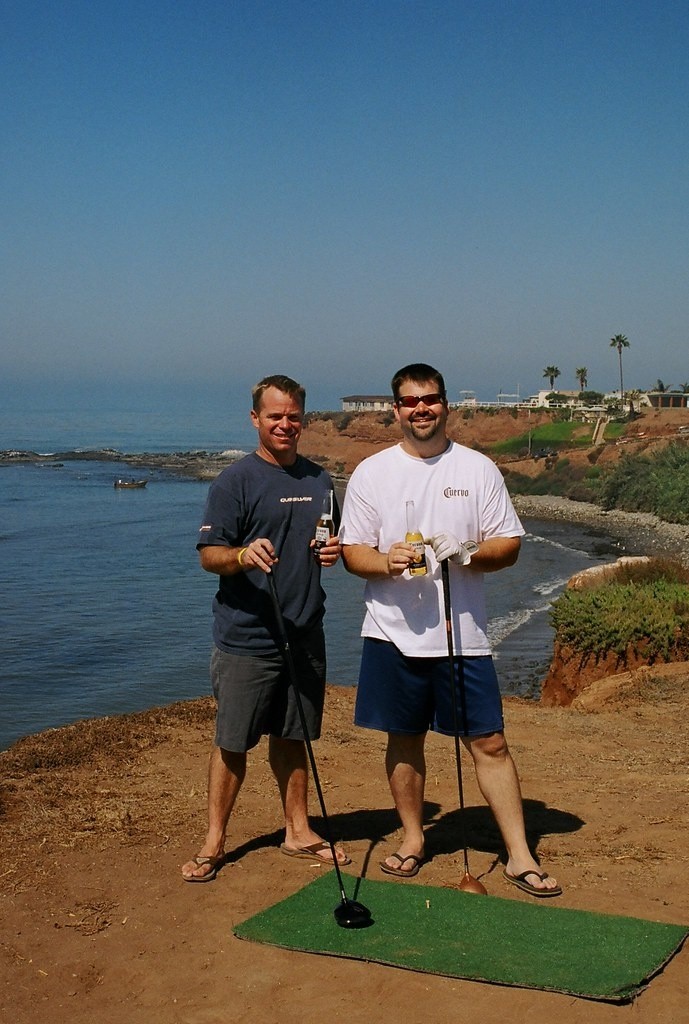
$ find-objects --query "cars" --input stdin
[531,448,557,458]
[676,426,689,434]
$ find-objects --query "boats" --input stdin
[113,479,148,488]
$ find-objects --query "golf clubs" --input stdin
[440,557,489,896]
[265,566,372,928]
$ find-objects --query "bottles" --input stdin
[313,489,335,560]
[405,501,428,577]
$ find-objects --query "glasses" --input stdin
[398,393,443,408]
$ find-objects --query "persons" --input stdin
[182,375,351,881]
[338,363,561,897]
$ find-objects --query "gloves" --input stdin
[424,532,479,565]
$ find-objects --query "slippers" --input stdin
[281,841,351,866]
[502,869,562,895]
[182,854,225,881]
[381,853,428,877]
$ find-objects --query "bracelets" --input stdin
[238,548,246,565]
